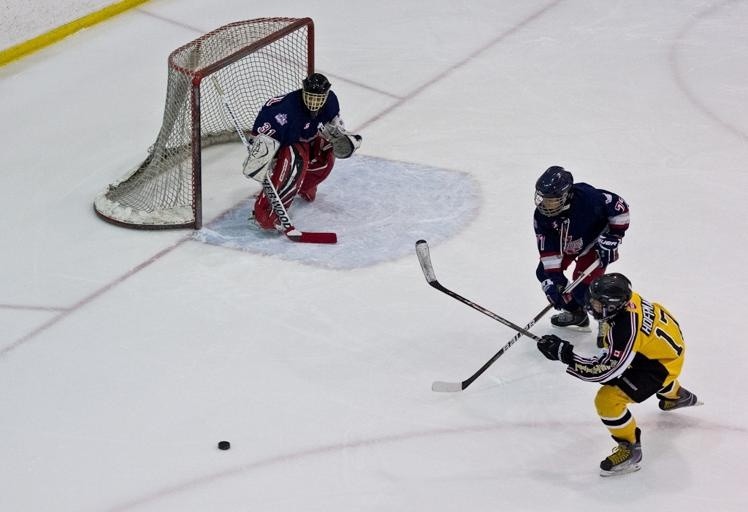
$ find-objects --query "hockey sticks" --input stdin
[432,260,597,393]
[416,240,542,344]
[211,75,338,245]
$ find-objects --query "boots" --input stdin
[659,386,696,410]
[600,428,643,471]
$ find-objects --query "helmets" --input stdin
[534,167,573,217]
[302,73,331,112]
[589,273,631,323]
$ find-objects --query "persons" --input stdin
[532,166,628,328]
[536,273,697,471]
[249,73,345,230]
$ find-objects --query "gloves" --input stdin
[540,277,573,310]
[590,232,622,268]
[537,334,573,364]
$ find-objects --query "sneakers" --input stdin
[551,311,589,326]
